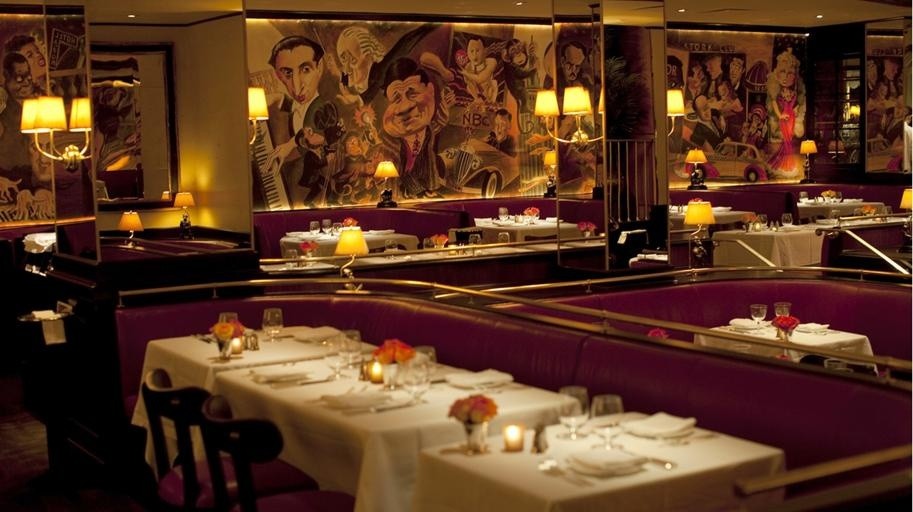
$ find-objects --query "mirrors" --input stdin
[604,0,669,273]
[864,18,909,173]
[551,0,607,274]
[44,6,99,265]
[91,41,179,211]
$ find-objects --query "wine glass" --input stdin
[782,214,793,228]
[750,302,767,325]
[498,208,508,222]
[470,234,481,257]
[285,250,297,270]
[423,237,435,248]
[758,214,768,231]
[774,301,791,321]
[799,191,807,204]
[558,386,589,445]
[404,357,429,405]
[592,394,624,451]
[534,212,540,223]
[217,312,238,326]
[310,221,320,236]
[321,219,332,235]
[337,330,361,375]
[263,308,284,344]
[498,232,509,243]
[835,192,842,203]
[384,240,397,261]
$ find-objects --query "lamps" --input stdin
[800,140,818,183]
[581,84,606,143]
[117,211,144,250]
[17,92,77,172]
[67,97,93,171]
[174,193,196,242]
[373,161,399,208]
[246,88,269,144]
[685,202,715,268]
[161,191,171,200]
[667,89,685,137]
[684,148,708,190]
[534,86,591,145]
[333,226,371,295]
[899,189,913,256]
[542,151,557,197]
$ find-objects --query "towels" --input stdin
[618,228,647,245]
[546,215,559,224]
[31,309,68,345]
[252,365,309,382]
[796,320,829,336]
[368,227,395,234]
[782,225,802,231]
[624,410,698,438]
[474,216,493,226]
[277,319,341,343]
[629,252,670,267]
[323,381,393,412]
[22,229,58,253]
[798,198,814,205]
[730,317,758,330]
[843,198,864,203]
[815,218,839,225]
[442,368,514,388]
[286,231,309,237]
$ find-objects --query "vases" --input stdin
[528,217,535,224]
[462,423,487,452]
[381,365,399,386]
[219,342,237,359]
[583,231,591,237]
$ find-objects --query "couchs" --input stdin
[248,208,466,259]
[401,196,557,239]
[733,184,910,213]
[665,186,797,233]
[485,278,912,368]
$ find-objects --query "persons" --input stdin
[675,46,809,178]
[93,95,147,198]
[0,34,59,221]
[866,59,912,158]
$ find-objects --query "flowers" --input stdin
[578,221,595,232]
[521,207,540,217]
[429,234,448,245]
[342,217,359,226]
[447,393,497,422]
[372,339,416,364]
[210,319,247,340]
[299,241,318,252]
[117,292,913,512]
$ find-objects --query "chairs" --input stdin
[141,369,319,512]
[198,391,357,512]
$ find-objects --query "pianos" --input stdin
[250,118,291,211]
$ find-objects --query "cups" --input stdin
[415,346,437,374]
